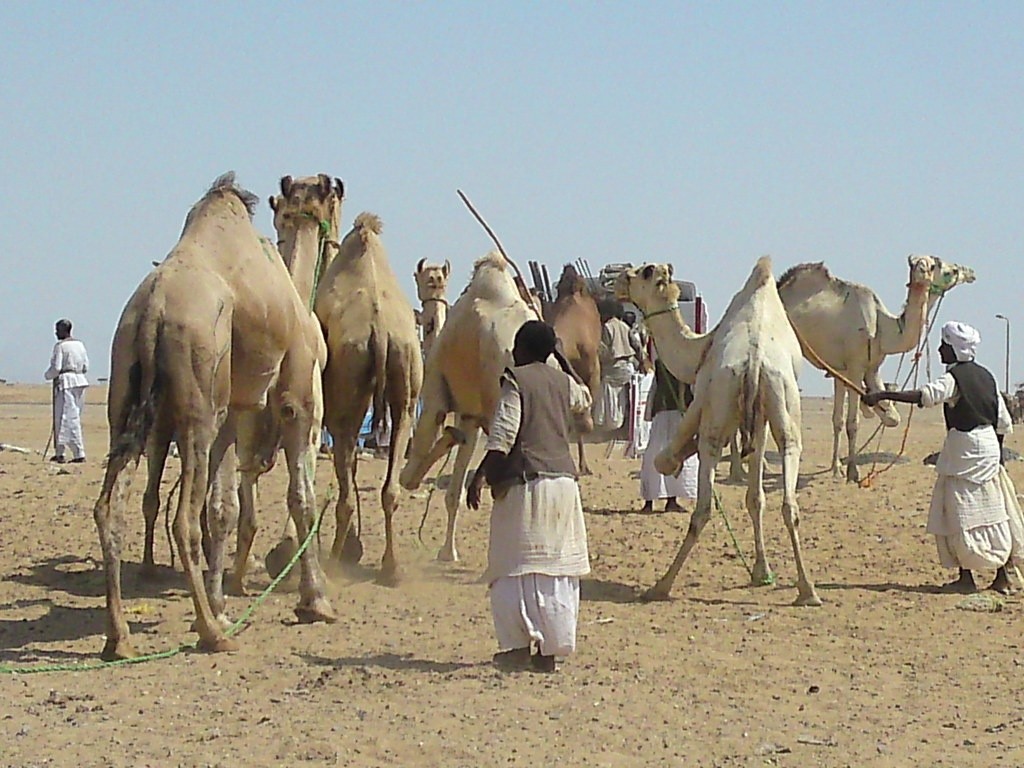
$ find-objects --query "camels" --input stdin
[616,249,978,607]
[92,169,602,663]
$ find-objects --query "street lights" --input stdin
[996,314,1009,394]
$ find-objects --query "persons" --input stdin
[858,319,1024,596]
[637,362,700,513]
[43,317,89,466]
[464,317,597,673]
[598,297,646,441]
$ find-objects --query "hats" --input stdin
[941,322,979,361]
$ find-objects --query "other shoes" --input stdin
[68,458,85,463]
[987,574,1008,592]
[640,505,652,512]
[943,573,976,592]
[50,456,65,463]
[666,503,688,512]
[494,644,555,673]
